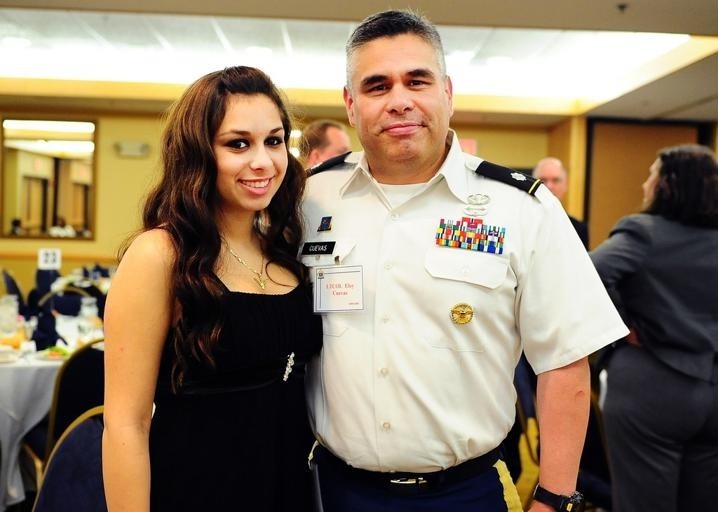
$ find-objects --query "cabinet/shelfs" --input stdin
[78,297,99,341]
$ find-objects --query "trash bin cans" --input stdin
[531,483,586,512]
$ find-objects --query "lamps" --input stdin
[0,264,113,512]
[532,381,617,512]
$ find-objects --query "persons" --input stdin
[295,118,354,172]
[576,142,716,509]
[278,4,634,509]
[531,157,590,252]
[98,64,327,510]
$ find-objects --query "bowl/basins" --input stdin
[216,230,268,290]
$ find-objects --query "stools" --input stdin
[390,473,426,491]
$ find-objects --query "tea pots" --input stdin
[1,115,99,240]
